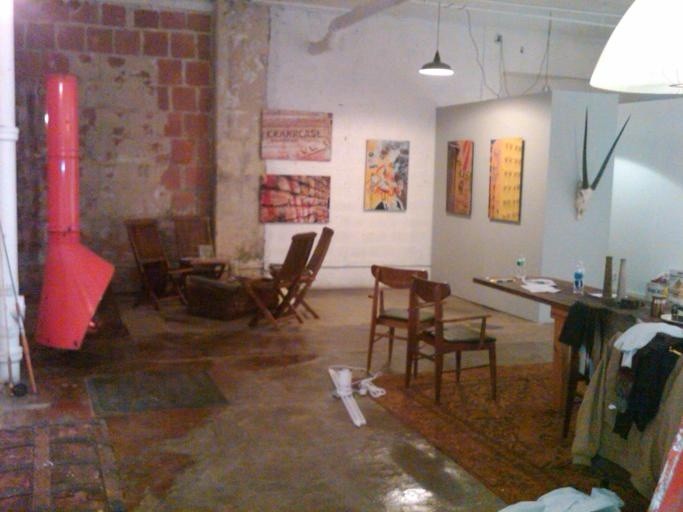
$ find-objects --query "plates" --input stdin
[659,313,682,324]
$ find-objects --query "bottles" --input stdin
[573,260,585,295]
[513,259,525,288]
[615,257,626,303]
[601,256,613,302]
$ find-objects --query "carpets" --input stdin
[346,361,651,512]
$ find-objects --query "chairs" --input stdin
[406,273,499,404]
[555,303,643,436]
[364,264,437,379]
[123,212,334,332]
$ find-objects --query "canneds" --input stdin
[651,295,667,318]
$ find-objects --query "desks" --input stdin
[472,273,681,421]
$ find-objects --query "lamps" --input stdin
[586,0,683,95]
[417,0,455,78]
